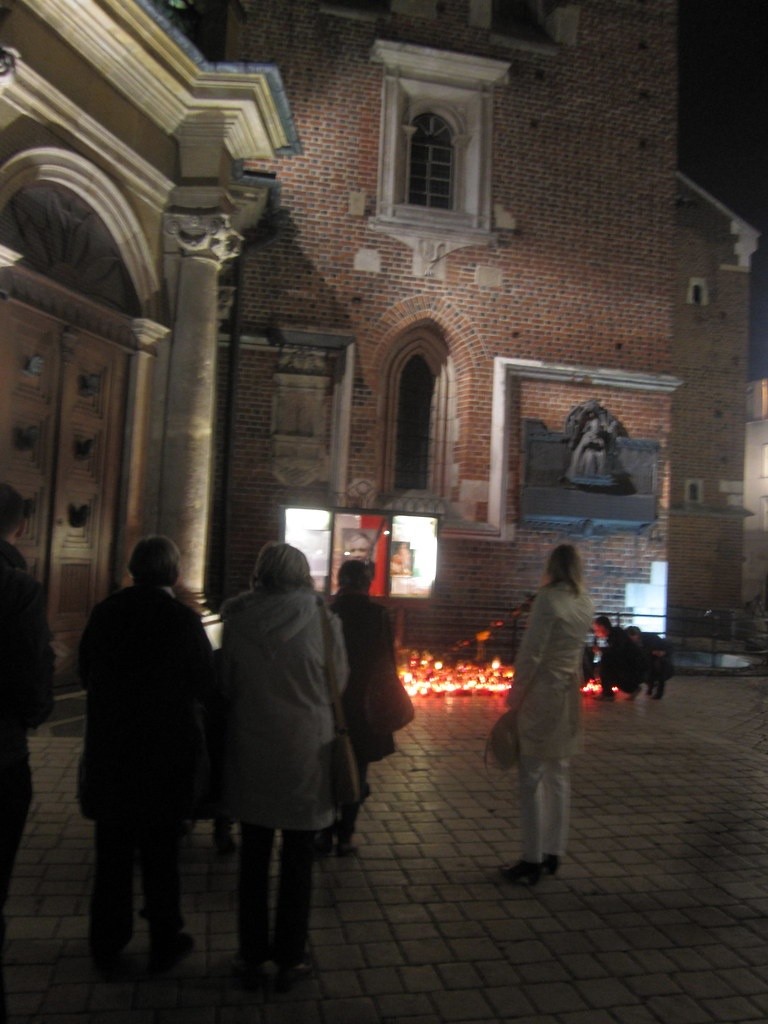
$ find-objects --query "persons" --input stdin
[592,619,648,701]
[348,534,374,560]
[312,560,415,859]
[493,543,593,886]
[627,626,674,698]
[0,481,58,1023]
[570,411,619,477]
[218,541,351,992]
[75,534,235,979]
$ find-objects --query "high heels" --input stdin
[542,854,559,874]
[501,859,542,886]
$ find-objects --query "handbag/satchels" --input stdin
[332,733,360,805]
[488,709,519,768]
[368,676,414,732]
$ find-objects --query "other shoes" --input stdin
[594,691,615,702]
[150,927,194,972]
[647,681,654,695]
[230,950,263,983]
[316,835,333,853]
[627,687,642,701]
[179,822,193,848]
[653,686,666,700]
[87,935,122,965]
[275,952,306,992]
[336,843,359,858]
[212,830,236,853]
